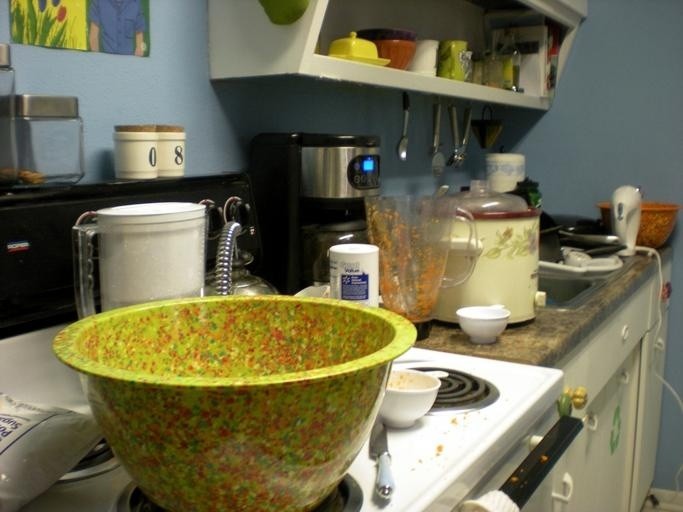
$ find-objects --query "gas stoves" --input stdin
[0,345,565,512]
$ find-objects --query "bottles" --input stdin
[1,42,86,193]
[496,27,522,93]
[113,123,187,180]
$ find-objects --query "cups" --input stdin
[362,195,477,343]
[70,200,211,322]
[405,39,440,76]
[438,39,469,83]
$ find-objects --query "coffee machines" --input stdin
[247,131,381,294]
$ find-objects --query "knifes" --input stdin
[369,420,394,501]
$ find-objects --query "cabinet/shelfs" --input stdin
[478,259,672,512]
[207,0,589,116]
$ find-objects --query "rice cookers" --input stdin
[418,179,548,326]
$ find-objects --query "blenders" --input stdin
[610,185,643,257]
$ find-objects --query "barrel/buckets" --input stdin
[88,200,207,310]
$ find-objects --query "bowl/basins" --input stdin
[563,251,592,268]
[378,367,442,429]
[595,202,682,250]
[456,305,511,346]
[52,294,418,512]
[356,28,418,71]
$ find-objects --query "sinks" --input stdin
[540,273,604,309]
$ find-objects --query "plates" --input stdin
[326,55,391,67]
